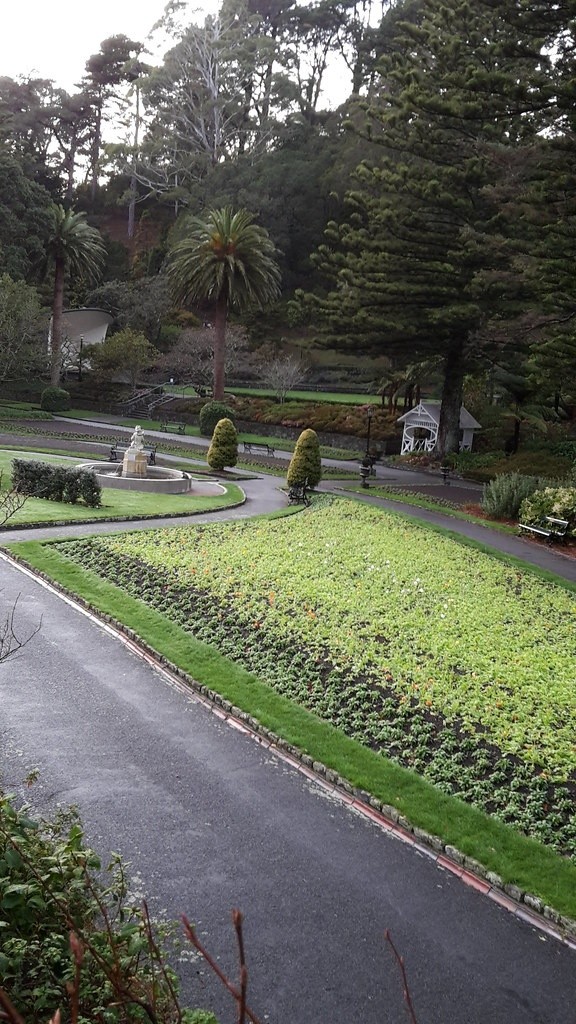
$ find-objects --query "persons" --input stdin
[130,424,144,451]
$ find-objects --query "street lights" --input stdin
[78,333,84,381]
[365,406,373,458]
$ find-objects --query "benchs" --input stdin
[517,515,570,548]
[244,442,275,458]
[160,421,186,435]
[107,441,157,465]
